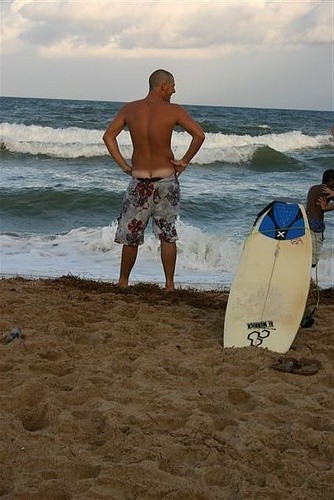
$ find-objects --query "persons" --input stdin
[103,68,206,293]
[306,169,334,268]
[316,187,334,210]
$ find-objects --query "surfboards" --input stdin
[223,201,312,353]
